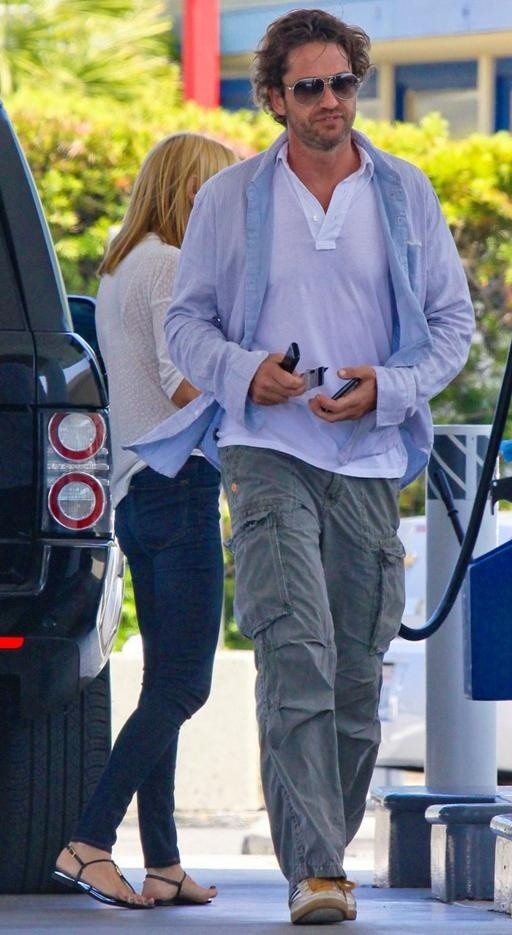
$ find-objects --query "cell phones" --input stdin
[325,377,361,413]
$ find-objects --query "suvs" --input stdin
[0,99,129,893]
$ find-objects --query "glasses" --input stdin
[284,72,359,105]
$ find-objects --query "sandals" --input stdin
[140,862,219,908]
[48,839,155,909]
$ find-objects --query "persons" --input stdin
[120,7,480,927]
[49,132,241,912]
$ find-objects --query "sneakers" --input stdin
[286,876,359,929]
